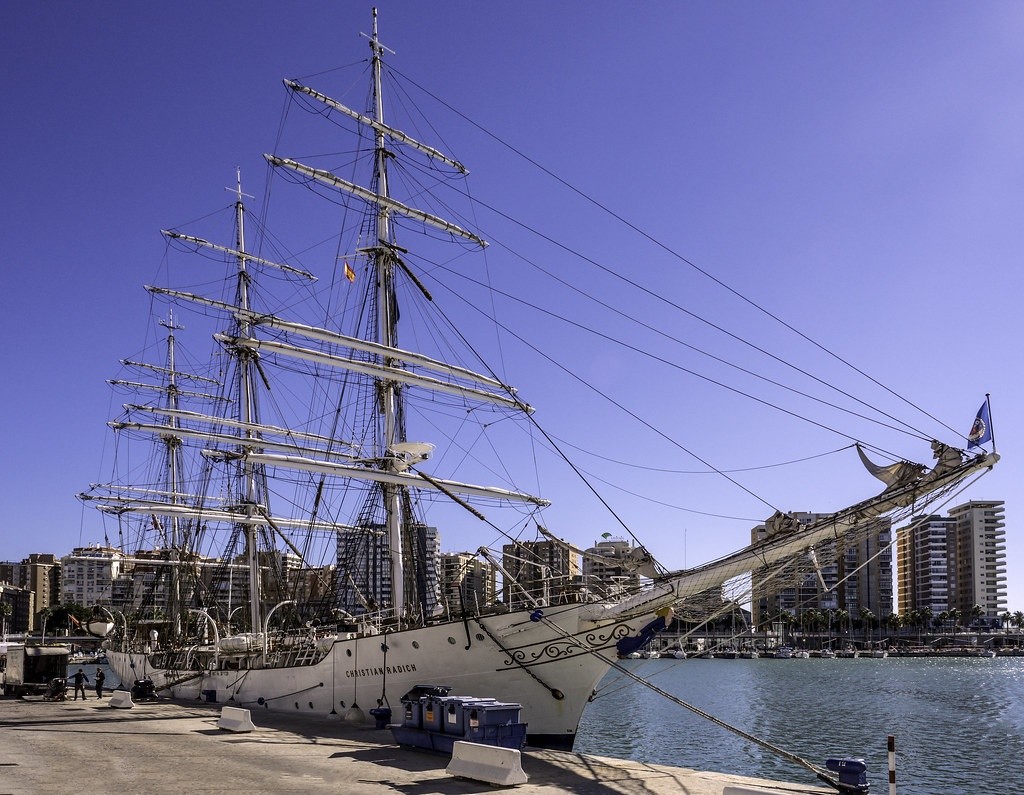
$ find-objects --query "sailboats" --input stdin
[71,3,1001,752]
[622,582,1024,662]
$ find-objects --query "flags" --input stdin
[966,400,993,450]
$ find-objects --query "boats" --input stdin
[66,614,116,637]
[383,684,529,754]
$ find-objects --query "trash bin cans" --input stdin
[443,697,496,737]
[419,696,473,732]
[401,685,452,729]
[462,702,524,737]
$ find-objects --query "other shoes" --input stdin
[83,698,87,700]
[74,698,77,701]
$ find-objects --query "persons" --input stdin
[67,668,90,701]
[93,667,105,700]
